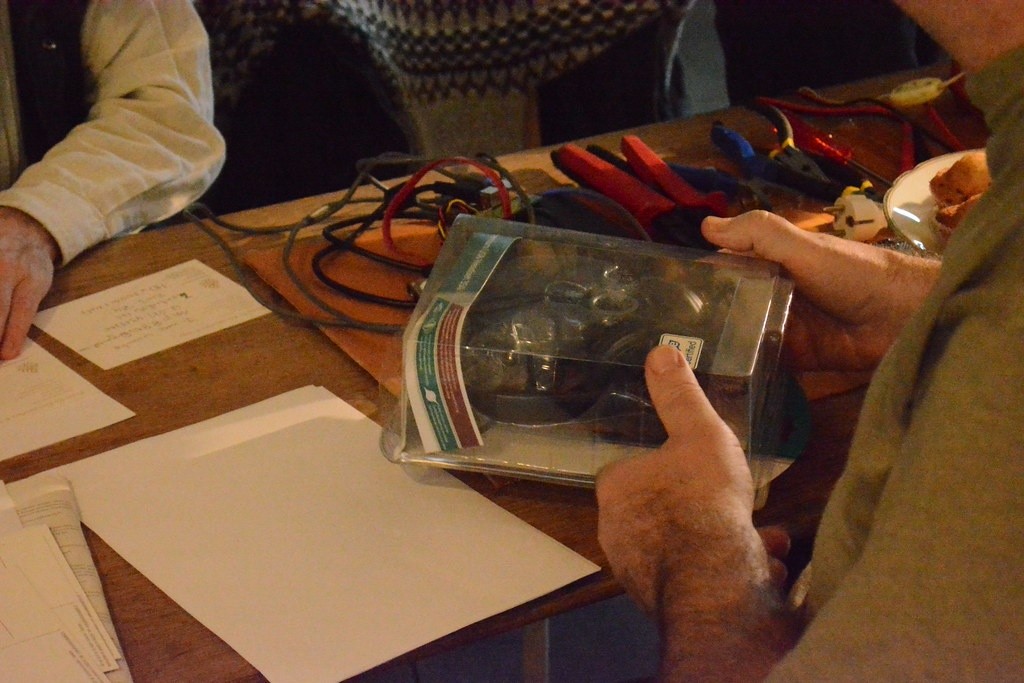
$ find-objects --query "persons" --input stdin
[0,0,228,362]
[592,1,1024,682]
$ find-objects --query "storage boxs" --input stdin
[378,213,814,488]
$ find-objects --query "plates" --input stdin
[884,149,994,258]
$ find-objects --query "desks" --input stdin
[0,57,1021,681]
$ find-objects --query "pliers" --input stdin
[749,97,833,186]
[815,156,886,204]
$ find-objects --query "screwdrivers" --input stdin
[765,108,894,188]
